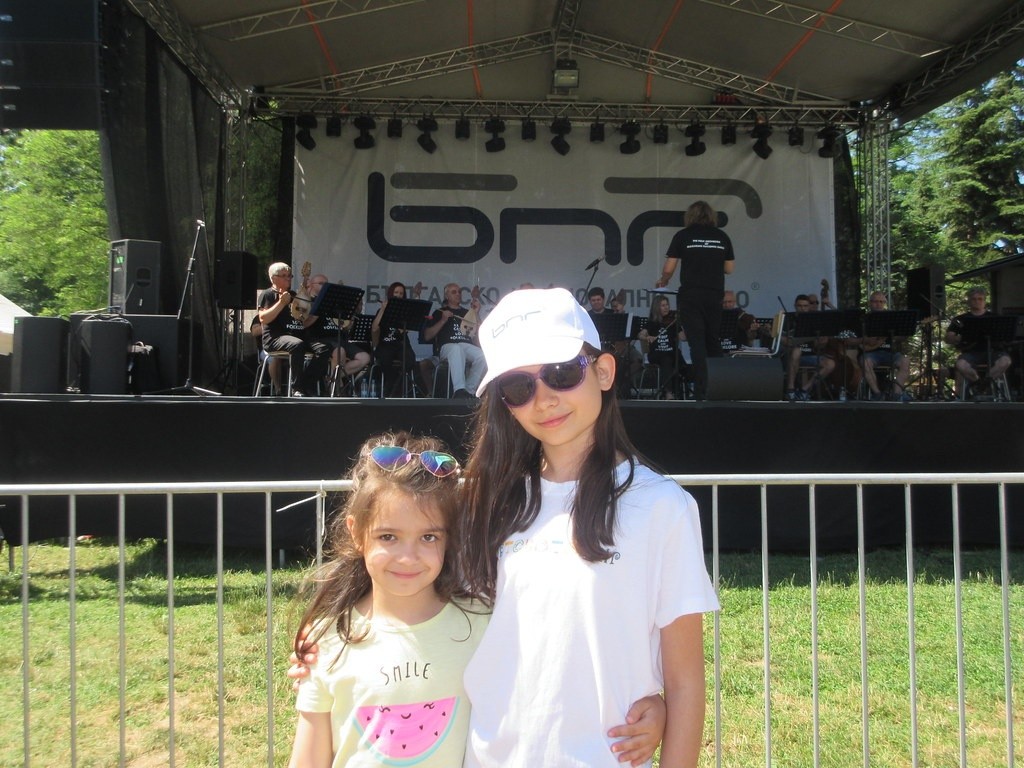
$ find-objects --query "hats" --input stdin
[476,287,601,399]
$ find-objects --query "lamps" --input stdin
[295,114,842,158]
[552,59,579,87]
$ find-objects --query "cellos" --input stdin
[819,279,861,393]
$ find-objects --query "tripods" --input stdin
[793,309,1016,401]
[202,309,271,395]
[644,287,696,401]
[309,281,365,397]
[142,220,222,395]
[378,299,434,398]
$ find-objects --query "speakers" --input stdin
[695,357,784,401]
[906,265,946,320]
[10,240,202,394]
[218,252,257,309]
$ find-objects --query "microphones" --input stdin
[584,256,604,271]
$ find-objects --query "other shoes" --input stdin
[792,387,806,400]
[870,391,882,400]
[895,393,911,401]
[454,389,473,398]
[293,387,307,396]
[784,389,795,399]
[969,383,981,395]
[688,393,705,400]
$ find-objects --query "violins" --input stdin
[664,309,684,326]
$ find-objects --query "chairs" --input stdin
[796,364,1009,402]
[255,339,660,399]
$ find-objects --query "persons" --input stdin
[289,431,667,768]
[944,286,1012,395]
[249,262,911,401]
[655,202,736,400]
[288,288,720,768]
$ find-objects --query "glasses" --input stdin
[447,290,461,294]
[312,282,323,285]
[495,356,596,406]
[369,445,463,478]
[273,274,293,280]
